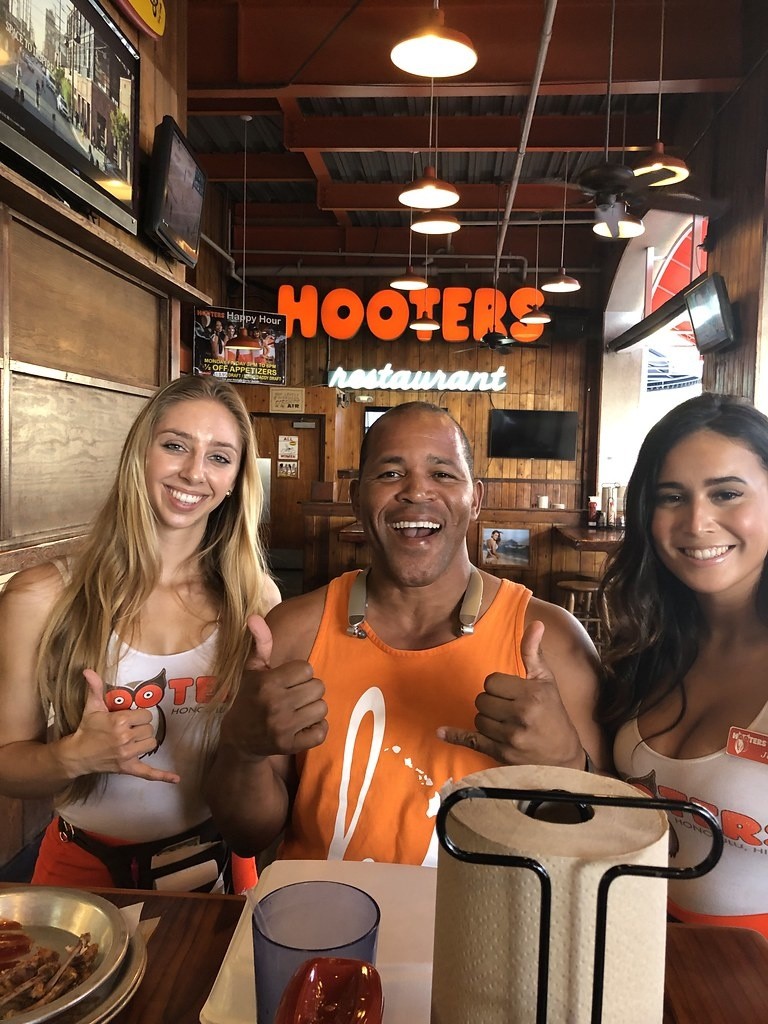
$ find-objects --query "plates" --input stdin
[200,859,438,1024]
[0,886,147,1024]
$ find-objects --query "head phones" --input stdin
[345,562,483,639]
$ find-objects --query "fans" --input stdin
[515,0,732,240]
[451,177,550,355]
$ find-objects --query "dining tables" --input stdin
[0,882,768,1024]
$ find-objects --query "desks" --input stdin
[554,523,625,560]
[338,519,370,543]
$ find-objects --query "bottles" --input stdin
[599,512,608,530]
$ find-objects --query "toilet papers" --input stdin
[428,763,671,1024]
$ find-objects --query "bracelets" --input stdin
[584,749,594,773]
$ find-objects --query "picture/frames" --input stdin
[277,460,300,479]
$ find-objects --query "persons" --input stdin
[486,530,501,564]
[202,401,605,880]
[210,320,276,364]
[0,372,280,899]
[592,396,768,937]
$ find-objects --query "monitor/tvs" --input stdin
[489,408,578,461]
[142,115,207,267]
[0,1,140,235]
[683,271,736,356]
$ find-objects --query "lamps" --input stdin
[519,216,551,325]
[389,182,427,291]
[409,98,460,234]
[541,152,581,292]
[389,0,477,77]
[593,94,645,239]
[398,77,459,209]
[409,262,439,330]
[225,112,262,351]
[629,0,688,186]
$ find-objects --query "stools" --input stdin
[553,572,602,627]
[556,581,611,657]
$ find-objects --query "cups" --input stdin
[537,495,549,508]
[251,879,381,1024]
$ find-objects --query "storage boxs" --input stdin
[310,479,338,503]
